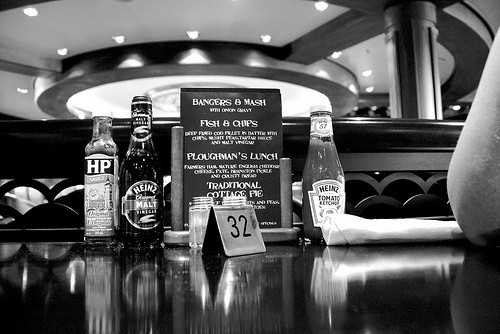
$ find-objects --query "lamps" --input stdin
[33,40,360,119]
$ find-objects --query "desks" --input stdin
[0,217,500,334]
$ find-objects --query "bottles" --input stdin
[187,197,214,249]
[82,110,121,254]
[119,94,166,251]
[221,196,247,206]
[301,106,346,242]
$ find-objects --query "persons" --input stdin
[444,25,500,243]
[448,251,500,334]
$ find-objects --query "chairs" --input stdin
[355,195,402,218]
[402,194,453,217]
[21,204,81,228]
